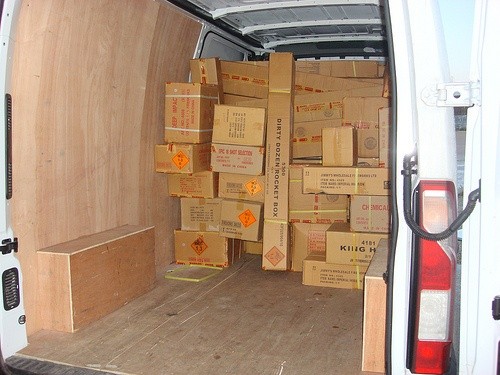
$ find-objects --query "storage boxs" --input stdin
[155,51,393,288]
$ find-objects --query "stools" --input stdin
[33,223,157,333]
[361,237,391,372]
